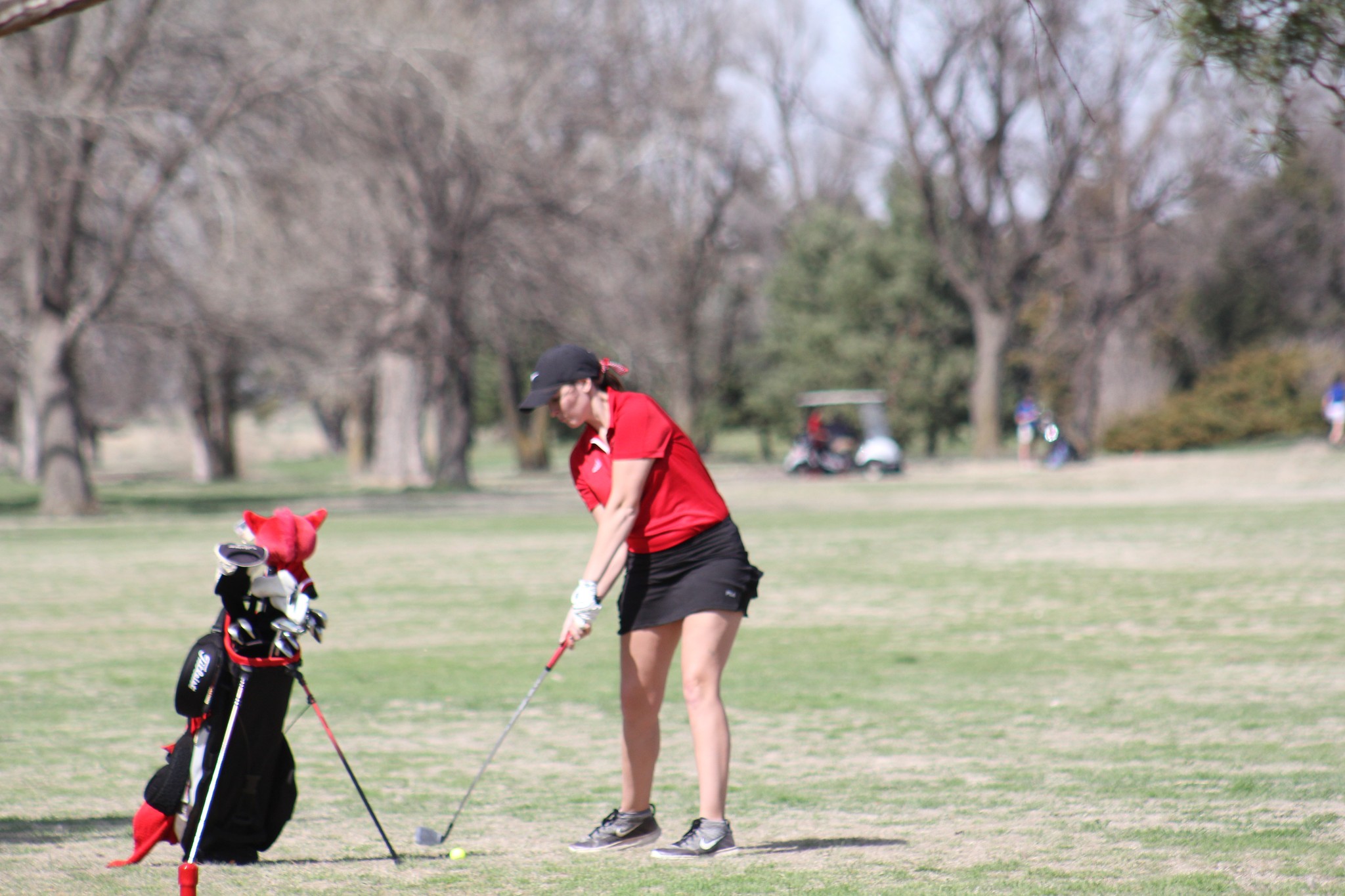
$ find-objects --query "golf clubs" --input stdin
[413,596,601,846]
[230,607,327,662]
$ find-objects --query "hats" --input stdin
[518,345,602,409]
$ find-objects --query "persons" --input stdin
[1322,373,1345,448]
[1013,389,1040,465]
[804,408,861,477]
[518,347,763,863]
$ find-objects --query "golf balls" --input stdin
[449,847,467,862]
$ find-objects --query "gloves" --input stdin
[571,579,602,628]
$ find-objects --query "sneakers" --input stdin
[568,803,661,853]
[650,817,739,859]
